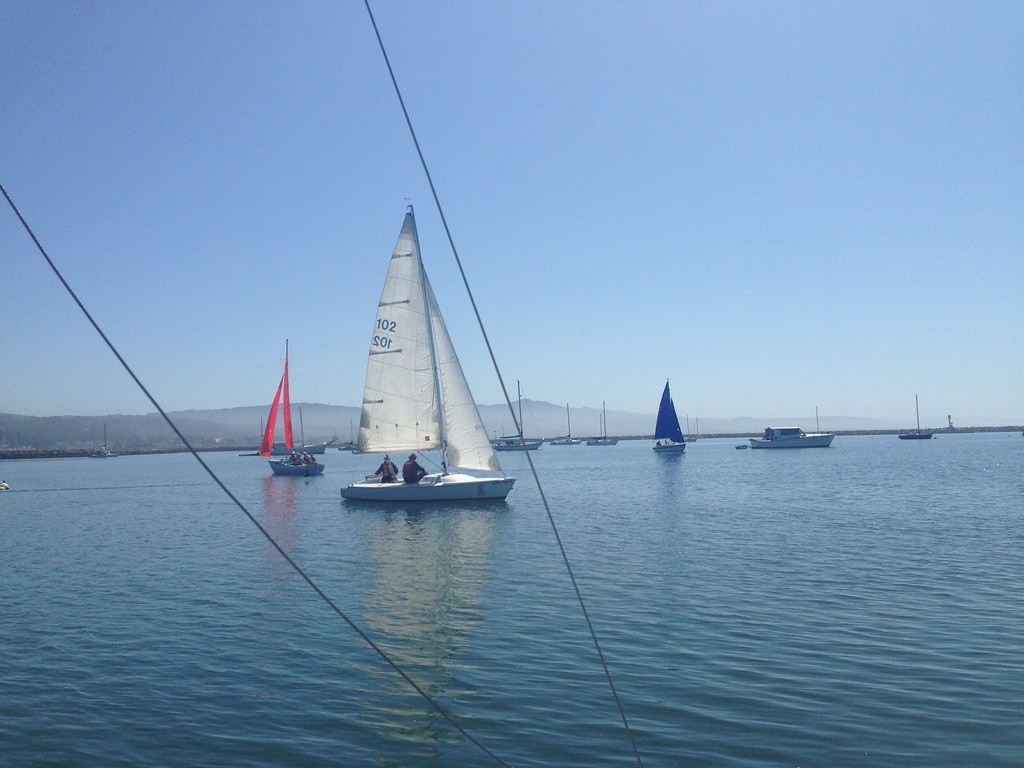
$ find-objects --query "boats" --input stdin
[749,427,836,449]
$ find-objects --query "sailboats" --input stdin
[340,204,517,503]
[586,401,619,445]
[550,403,582,445]
[270,408,326,455]
[898,394,933,440]
[653,378,686,452]
[493,381,543,451]
[685,415,697,441]
[260,339,325,476]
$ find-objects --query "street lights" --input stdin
[91,422,119,458]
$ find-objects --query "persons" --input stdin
[665,440,669,445]
[403,454,428,483]
[289,451,316,465]
[375,456,398,483]
[656,441,661,447]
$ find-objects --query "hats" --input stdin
[384,457,391,460]
[291,452,295,455]
[297,453,301,455]
[303,452,306,455]
[409,454,416,459]
